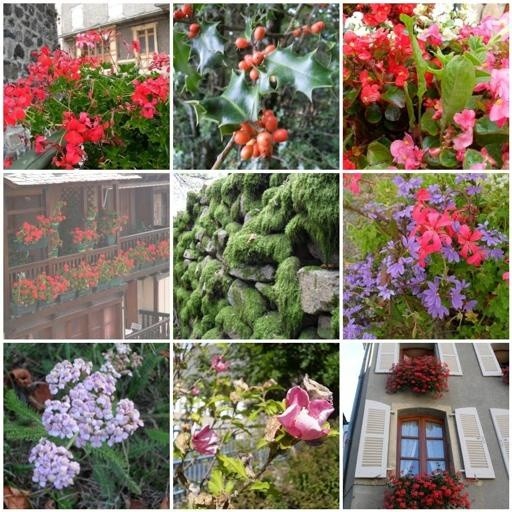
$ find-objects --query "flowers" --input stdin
[385,354,450,399]
[383,466,478,509]
[502,367,509,385]
[11,201,169,307]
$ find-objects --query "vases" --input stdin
[10,303,37,318]
